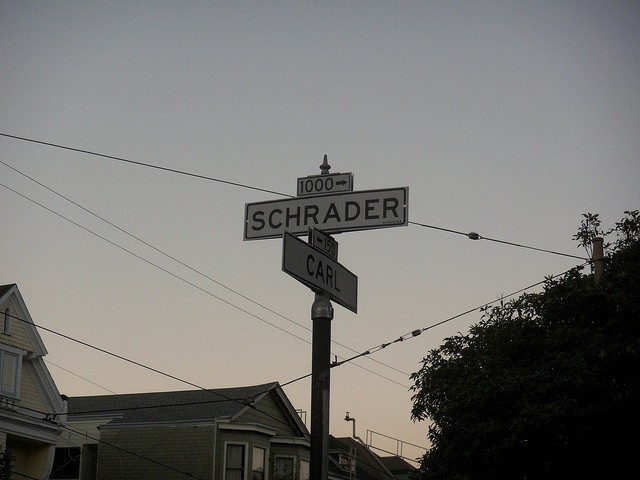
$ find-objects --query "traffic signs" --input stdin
[281,227,358,314]
[243,172,409,241]
